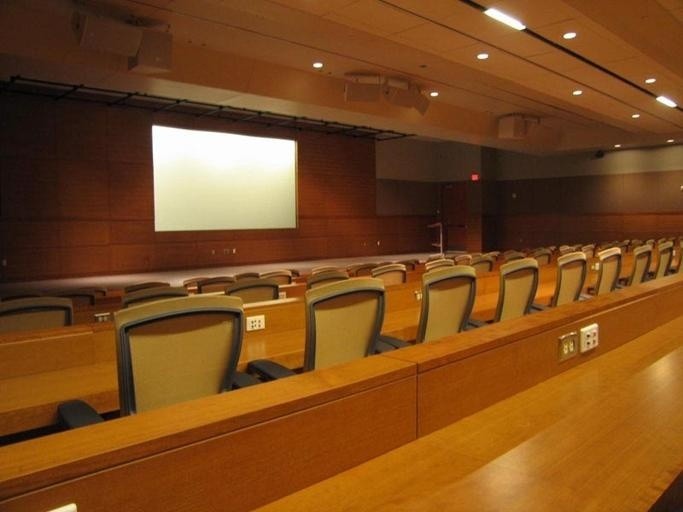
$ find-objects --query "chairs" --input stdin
[0,297,73,330]
[425,259,456,271]
[380,266,476,348]
[246,276,386,381]
[656,241,674,279]
[582,244,595,258]
[291,264,311,276]
[184,275,206,288]
[627,245,652,288]
[122,286,188,308]
[469,257,541,328]
[307,273,347,288]
[259,269,291,284]
[235,273,259,278]
[224,280,279,303]
[454,254,472,265]
[57,294,261,427]
[469,257,493,271]
[197,277,235,294]
[579,247,622,299]
[530,250,551,265]
[532,253,585,310]
[372,263,407,285]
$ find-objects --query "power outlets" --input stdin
[558,332,578,363]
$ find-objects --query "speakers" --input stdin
[66,12,145,57]
[128,17,172,75]
[497,114,527,141]
